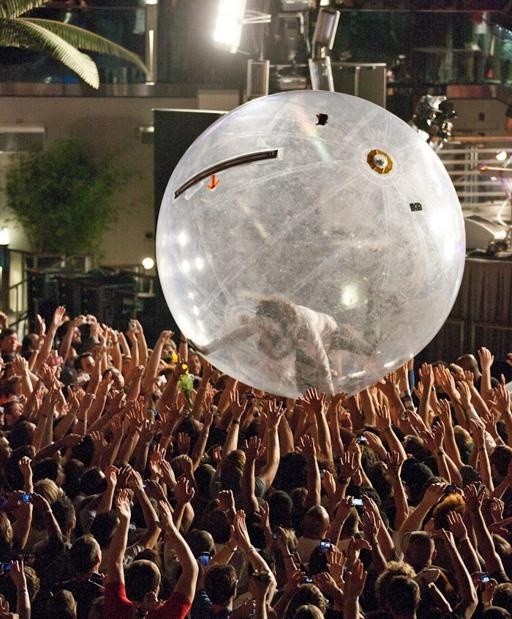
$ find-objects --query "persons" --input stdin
[504,102,512,137]
[0,298,511,618]
